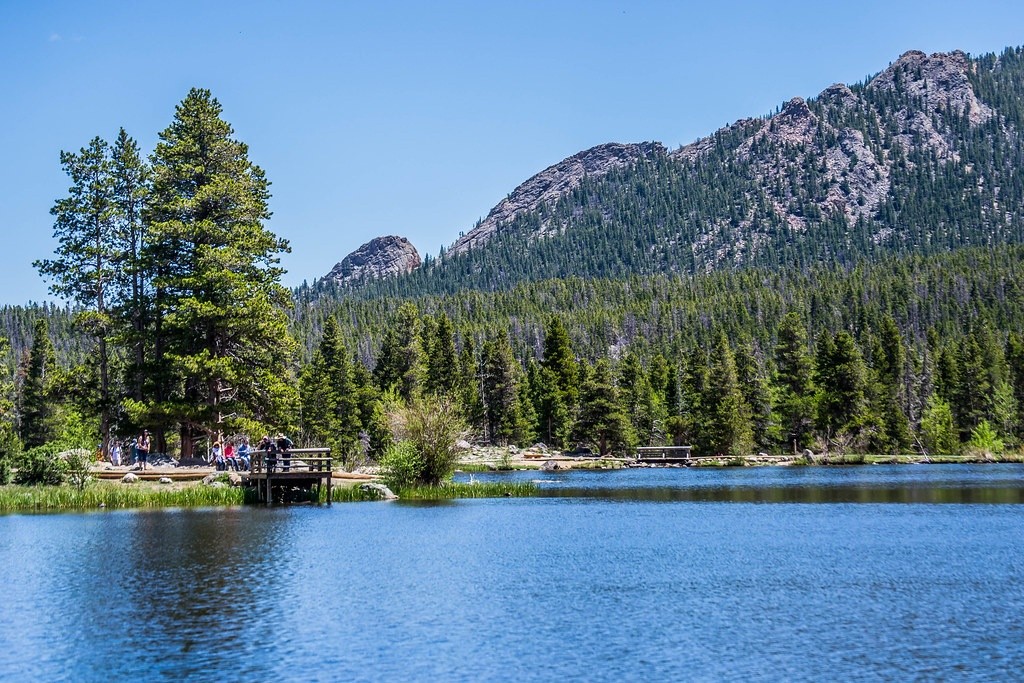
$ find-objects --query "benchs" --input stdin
[636,446,694,461]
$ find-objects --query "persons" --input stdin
[110,428,150,470]
[256,433,293,473]
[212,437,251,471]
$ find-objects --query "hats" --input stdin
[274,433,287,440]
[213,441,220,448]
